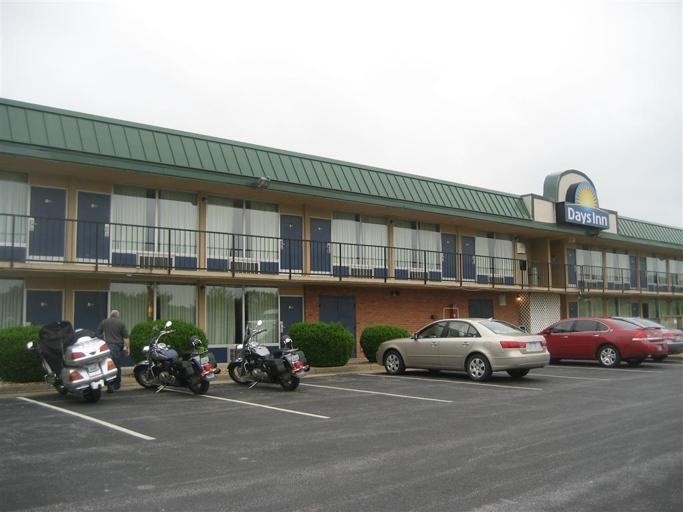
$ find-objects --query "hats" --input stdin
[536,317,682,366]
[375,318,550,382]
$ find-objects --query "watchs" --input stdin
[134,320,221,394]
[26,320,119,403]
[228,319,309,390]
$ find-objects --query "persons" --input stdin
[93,309,129,393]
